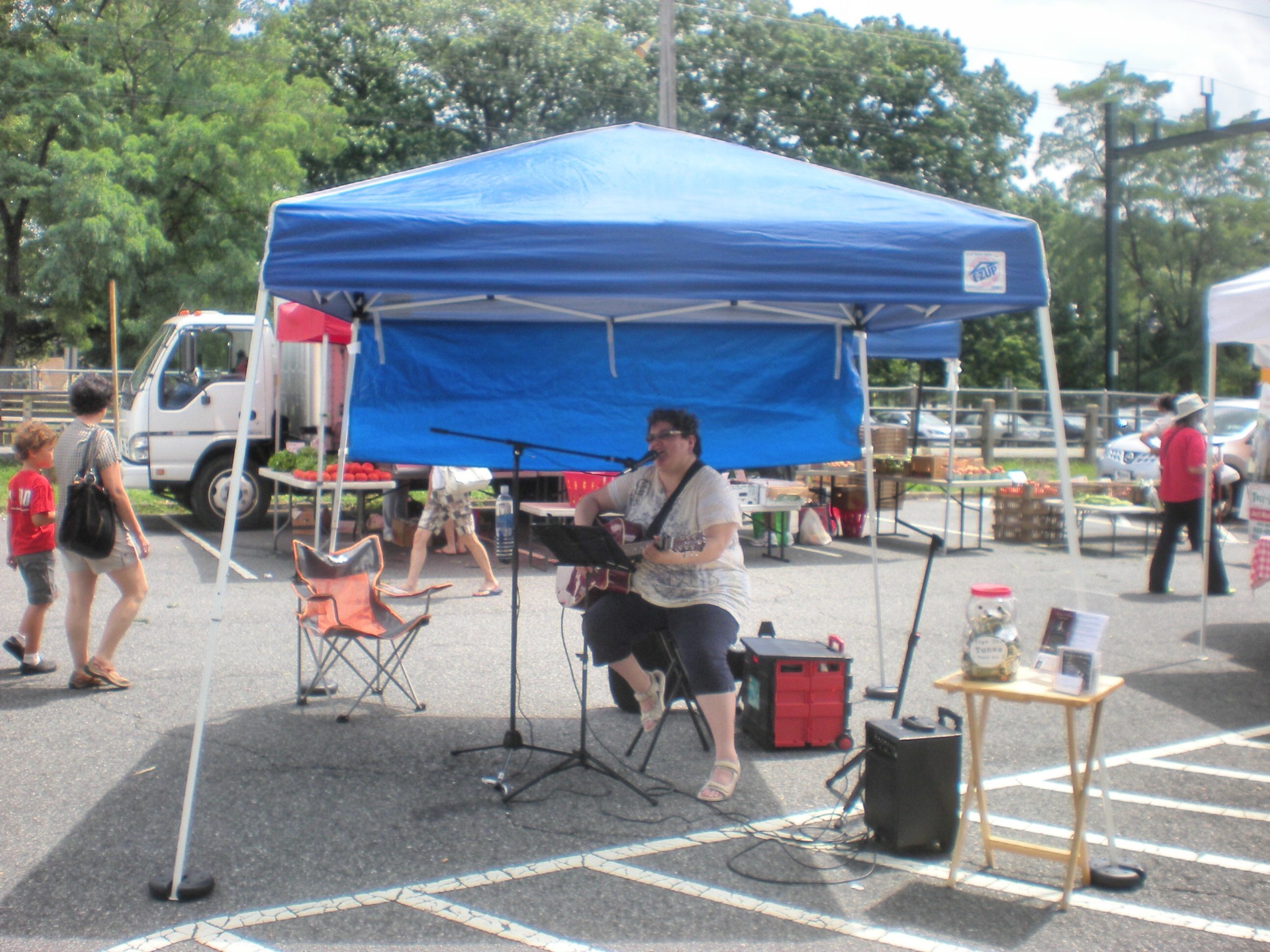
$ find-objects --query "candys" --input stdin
[962,606,1020,683]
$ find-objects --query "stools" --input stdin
[623,633,713,773]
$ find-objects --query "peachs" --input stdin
[950,464,1006,475]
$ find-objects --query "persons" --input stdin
[2,421,59,676]
[398,464,503,596]
[575,407,747,800]
[434,519,467,555]
[53,373,152,687]
[748,464,798,546]
[1140,391,1236,597]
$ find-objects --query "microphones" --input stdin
[618,450,658,476]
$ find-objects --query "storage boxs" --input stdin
[391,518,435,548]
[291,504,329,537]
[739,635,856,751]
[562,471,620,506]
[725,425,1146,546]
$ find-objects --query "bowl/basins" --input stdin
[875,454,912,474]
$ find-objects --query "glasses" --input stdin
[645,429,688,443]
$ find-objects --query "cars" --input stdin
[1029,411,1105,447]
[1117,404,1165,435]
[957,411,1056,447]
[1101,397,1262,526]
[874,409,969,449]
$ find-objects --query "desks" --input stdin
[859,472,1011,556]
[1042,499,1156,555]
[933,666,1125,908]
[521,502,624,571]
[740,505,801,562]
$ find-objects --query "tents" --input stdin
[168,120,1119,899]
[1198,266,1270,662]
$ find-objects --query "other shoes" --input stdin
[2,635,22,662]
[82,656,132,688]
[1206,587,1237,595]
[1148,587,1174,594]
[68,670,110,689]
[20,655,57,675]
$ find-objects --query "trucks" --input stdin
[116,295,575,534]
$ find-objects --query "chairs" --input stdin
[291,535,453,723]
[259,468,396,550]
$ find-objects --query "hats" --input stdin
[1170,393,1209,421]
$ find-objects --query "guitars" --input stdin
[553,514,707,611]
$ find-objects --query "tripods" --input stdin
[431,426,660,809]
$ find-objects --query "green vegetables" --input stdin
[268,447,339,473]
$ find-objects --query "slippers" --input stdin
[436,547,469,556]
[474,588,503,597]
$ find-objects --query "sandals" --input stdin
[697,760,741,801]
[634,670,666,732]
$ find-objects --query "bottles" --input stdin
[495,486,514,556]
[962,585,1021,682]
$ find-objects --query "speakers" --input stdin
[863,718,961,847]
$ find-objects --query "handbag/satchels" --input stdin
[58,426,117,560]
[439,465,492,495]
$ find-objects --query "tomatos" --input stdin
[293,462,391,482]
[998,480,1057,494]
